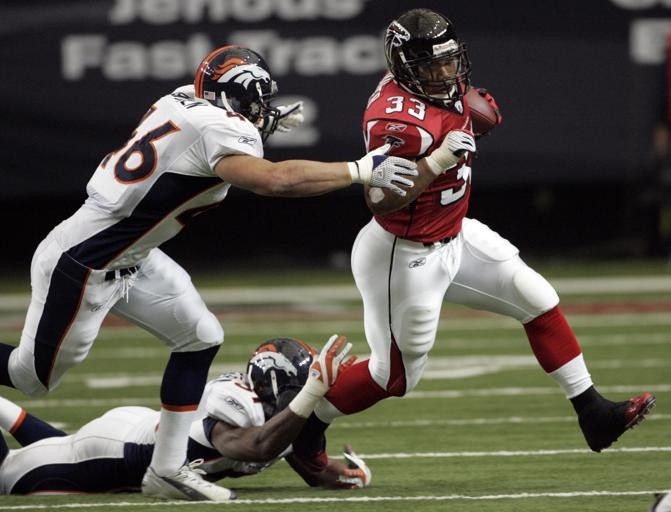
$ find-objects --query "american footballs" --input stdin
[464,86,496,131]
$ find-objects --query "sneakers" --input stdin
[276,391,328,473]
[142,457,236,501]
[580,391,656,453]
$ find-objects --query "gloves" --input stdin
[432,131,477,172]
[349,143,419,197]
[331,444,372,490]
[263,101,305,131]
[309,334,358,398]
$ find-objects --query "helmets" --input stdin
[245,338,319,407]
[383,8,471,100]
[194,46,281,130]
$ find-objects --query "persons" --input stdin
[293,9,655,475]
[0,334,371,497]
[0,45,418,502]
